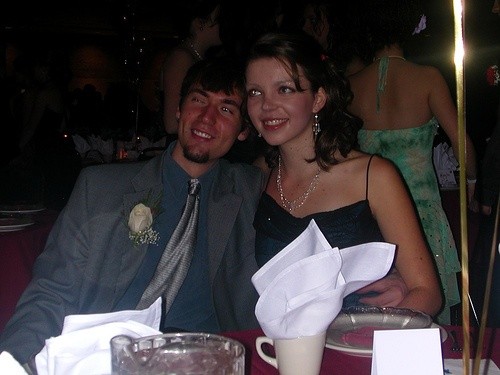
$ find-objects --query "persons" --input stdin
[345,0,476,326]
[0,58,408,375]
[161,0,223,134]
[240,32,445,332]
[301,0,346,58]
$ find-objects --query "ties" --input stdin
[134,180,202,317]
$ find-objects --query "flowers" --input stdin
[120,188,165,251]
[412,14,427,36]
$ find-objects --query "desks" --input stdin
[214,325,500,375]
[0,207,59,338]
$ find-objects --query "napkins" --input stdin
[34,295,166,375]
[250,219,396,339]
[444,358,500,375]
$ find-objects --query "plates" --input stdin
[324,313,448,357]
[0,207,46,216]
[325,306,432,349]
[0,219,34,232]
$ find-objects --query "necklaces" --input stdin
[374,55,406,61]
[276,157,320,212]
[188,41,201,60]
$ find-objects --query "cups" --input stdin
[256,337,325,375]
[111,332,245,375]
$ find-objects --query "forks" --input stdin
[450,330,462,353]
